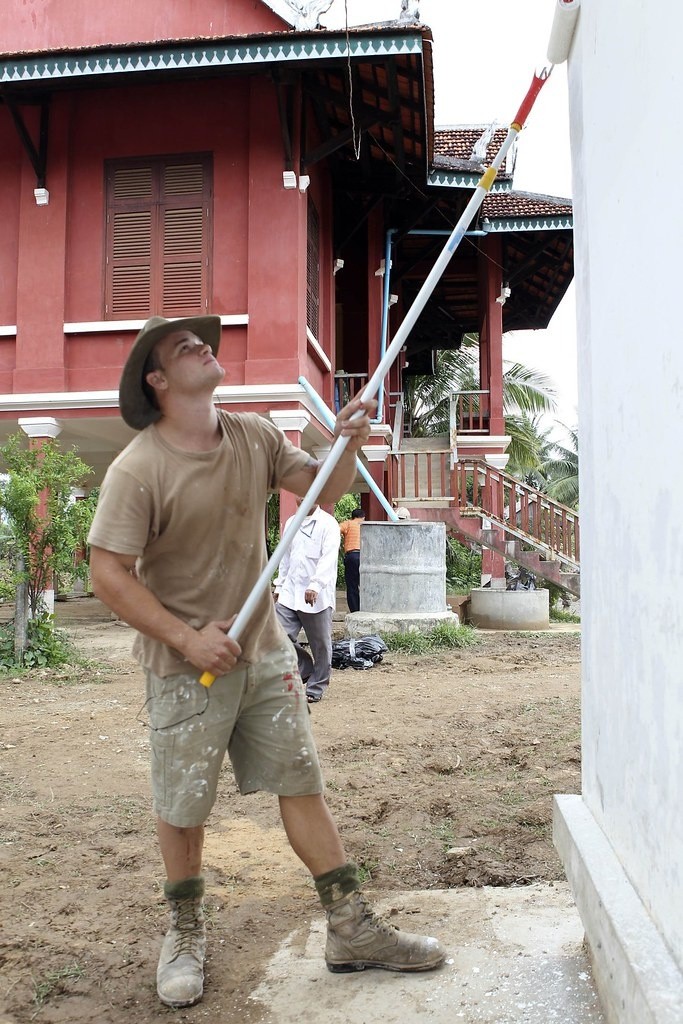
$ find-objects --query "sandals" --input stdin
[305,692,323,702]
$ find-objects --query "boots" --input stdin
[156,897,207,1006]
[324,888,445,973]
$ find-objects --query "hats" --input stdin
[119,315,221,431]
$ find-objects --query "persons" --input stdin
[272,496,342,704]
[85,314,449,1009]
[339,508,366,613]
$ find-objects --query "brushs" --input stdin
[197,0,587,694]
